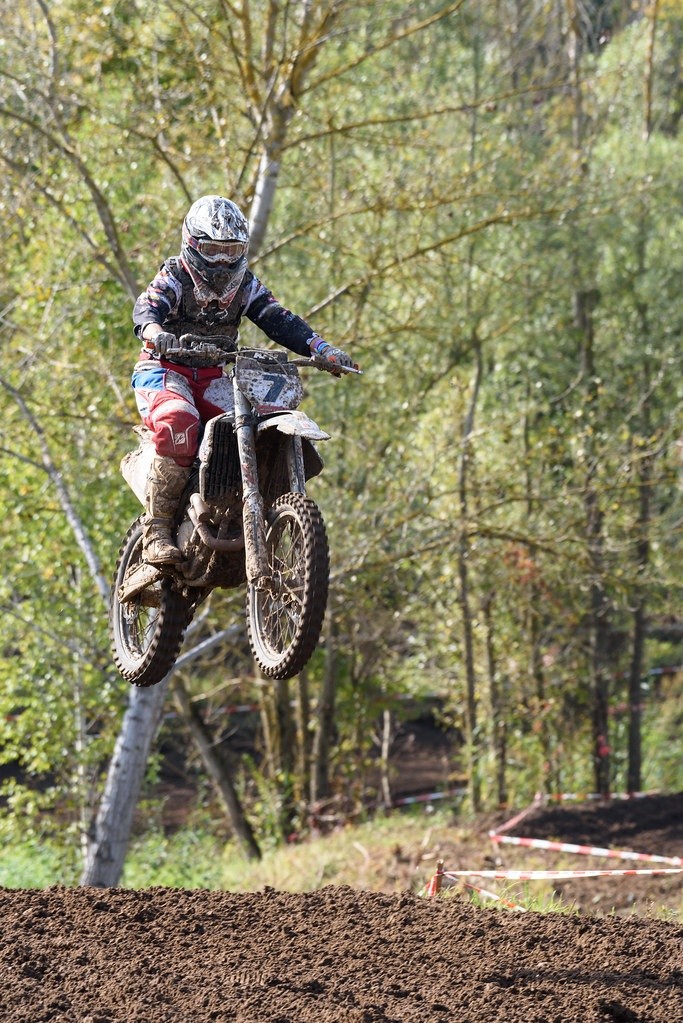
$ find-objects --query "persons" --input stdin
[132,195,354,564]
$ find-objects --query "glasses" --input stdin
[197,238,247,263]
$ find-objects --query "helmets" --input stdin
[182,195,249,291]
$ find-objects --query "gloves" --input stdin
[153,331,178,355]
[322,347,354,379]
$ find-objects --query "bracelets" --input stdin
[313,340,329,353]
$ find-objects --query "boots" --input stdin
[142,454,191,564]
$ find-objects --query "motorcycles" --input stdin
[108,331,364,690]
